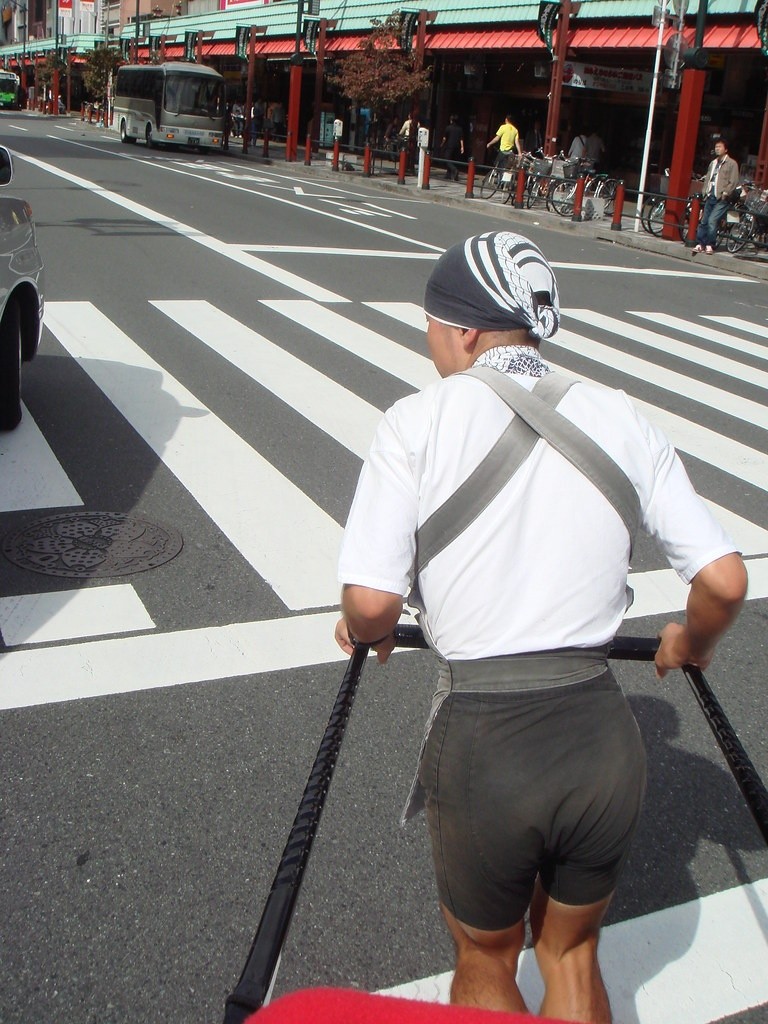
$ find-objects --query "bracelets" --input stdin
[347,627,389,648]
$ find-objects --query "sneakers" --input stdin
[692,244,703,253]
[705,246,714,256]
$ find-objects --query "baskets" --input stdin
[562,162,578,178]
[744,188,768,215]
[493,153,514,169]
[529,158,551,175]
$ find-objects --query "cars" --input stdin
[0,142,47,432]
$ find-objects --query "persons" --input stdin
[440,114,464,180]
[522,119,544,152]
[582,125,608,162]
[334,230,749,1024]
[253,98,265,139]
[384,112,429,151]
[567,127,588,157]
[230,103,254,138]
[692,137,740,255]
[487,114,522,168]
[271,102,284,141]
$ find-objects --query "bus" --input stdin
[0,71,19,110]
[116,62,226,152]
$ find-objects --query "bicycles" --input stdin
[482,150,768,258]
